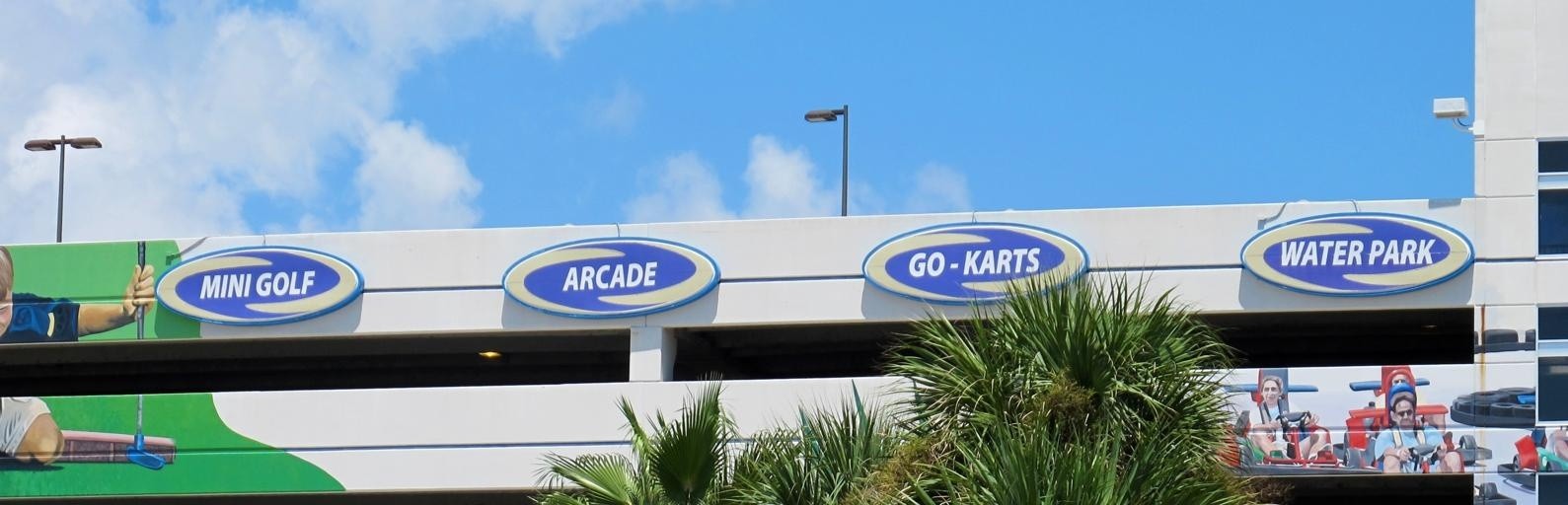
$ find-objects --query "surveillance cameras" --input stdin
[1432,96,1469,117]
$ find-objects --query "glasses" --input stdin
[1396,409,1412,416]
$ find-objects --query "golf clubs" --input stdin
[127,242,165,469]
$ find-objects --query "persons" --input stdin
[1371,368,1427,409]
[0,242,157,467]
[1541,425,1568,465]
[1248,375,1334,462]
[1373,390,1464,475]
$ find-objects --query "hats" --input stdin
[1387,369,1416,411]
[1261,376,1283,393]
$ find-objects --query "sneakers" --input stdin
[1269,441,1284,450]
[1308,444,1333,460]
